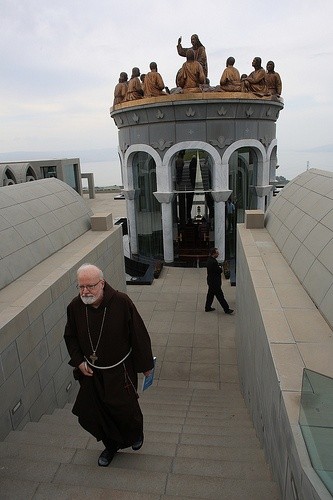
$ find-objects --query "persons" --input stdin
[227,198,234,232]
[143,62,169,98]
[177,49,210,88]
[240,57,282,98]
[190,155,196,183]
[177,34,207,77]
[63,262,155,467]
[219,57,242,92]
[176,154,184,183]
[114,67,146,105]
[204,248,233,314]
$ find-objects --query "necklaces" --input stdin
[86,305,106,365]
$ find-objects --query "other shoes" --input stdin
[206,308,215,312]
[226,309,233,313]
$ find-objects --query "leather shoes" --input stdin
[132,439,143,450]
[97,448,119,466]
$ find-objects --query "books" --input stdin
[142,356,156,391]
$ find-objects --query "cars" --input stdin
[274,185,284,192]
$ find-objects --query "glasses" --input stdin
[75,280,102,290]
